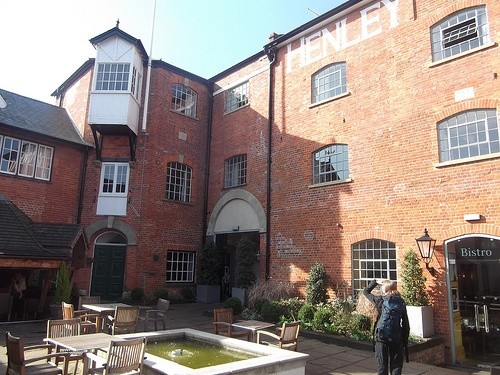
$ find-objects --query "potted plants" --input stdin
[402,248,434,339]
[48,262,72,317]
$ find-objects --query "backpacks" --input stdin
[378,296,401,338]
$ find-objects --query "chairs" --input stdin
[212,308,251,340]
[257,321,300,351]
[5,296,171,375]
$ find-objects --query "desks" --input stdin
[82,303,132,329]
[231,320,275,343]
[43,333,126,375]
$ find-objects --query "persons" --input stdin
[12,269,32,320]
[363,278,410,375]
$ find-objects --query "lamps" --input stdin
[416,229,437,274]
